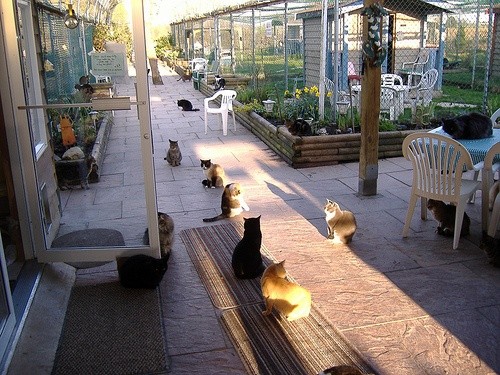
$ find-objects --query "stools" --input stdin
[56,160,89,190]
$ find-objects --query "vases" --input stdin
[296,117,313,129]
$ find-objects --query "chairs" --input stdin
[402,132,500,250]
[324,49,438,122]
[192,57,207,86]
[204,90,237,136]
[89,70,111,82]
[490,108,500,129]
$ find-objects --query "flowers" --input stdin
[284,86,333,118]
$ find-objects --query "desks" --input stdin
[412,126,500,170]
[352,85,407,116]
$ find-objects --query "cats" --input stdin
[322,198,358,245]
[426,198,471,236]
[199,158,225,191]
[176,73,193,82]
[213,75,225,91]
[56,154,96,191]
[177,99,200,111]
[75,75,95,93]
[202,182,250,223]
[287,119,311,137]
[444,113,494,140]
[143,212,176,255]
[163,138,183,167]
[260,259,312,320]
[232,214,264,279]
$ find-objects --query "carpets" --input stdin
[51,229,125,269]
[51,282,171,375]
[218,299,380,375]
[177,223,301,310]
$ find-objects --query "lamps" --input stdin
[262,99,276,112]
[64,4,79,29]
